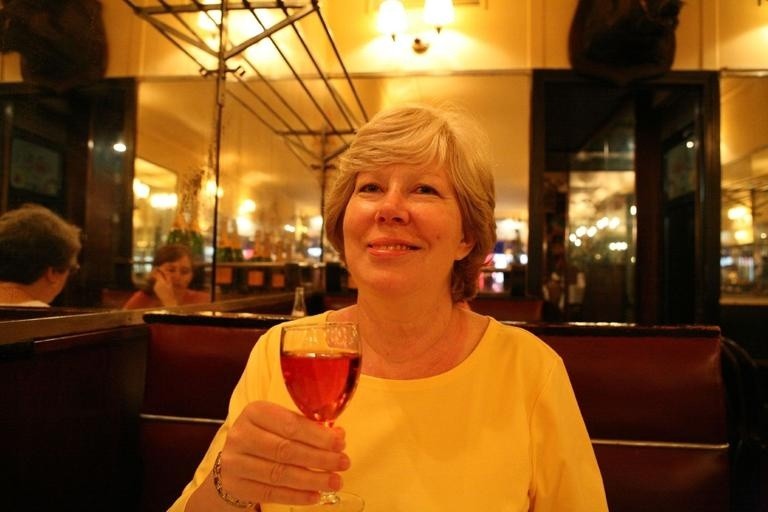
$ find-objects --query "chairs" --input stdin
[144,320,731,510]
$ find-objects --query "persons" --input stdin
[124,245,211,309]
[0,202,82,307]
[165,106,609,512]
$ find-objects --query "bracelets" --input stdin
[213,452,256,509]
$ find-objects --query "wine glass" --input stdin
[278,321,367,509]
